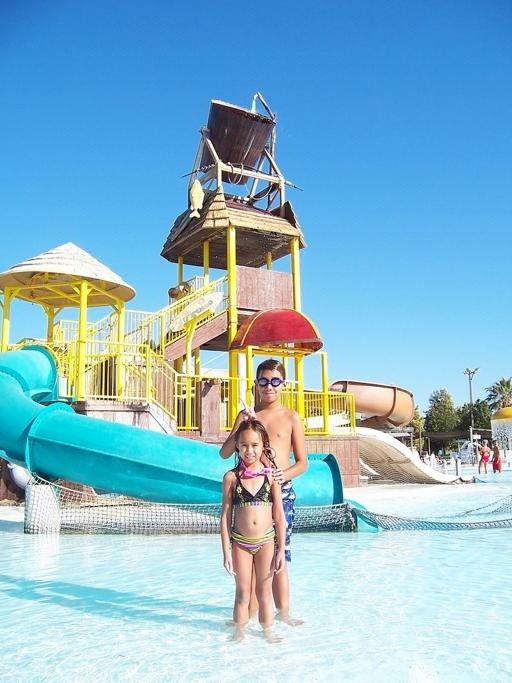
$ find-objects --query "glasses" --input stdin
[256,378,284,387]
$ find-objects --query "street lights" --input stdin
[464,368,480,428]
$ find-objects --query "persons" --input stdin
[418,448,447,470]
[220,418,286,644]
[219,359,308,629]
[473,434,509,453]
[490,440,502,474]
[478,439,490,475]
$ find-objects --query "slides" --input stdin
[0,345,377,533]
[305,379,461,484]
